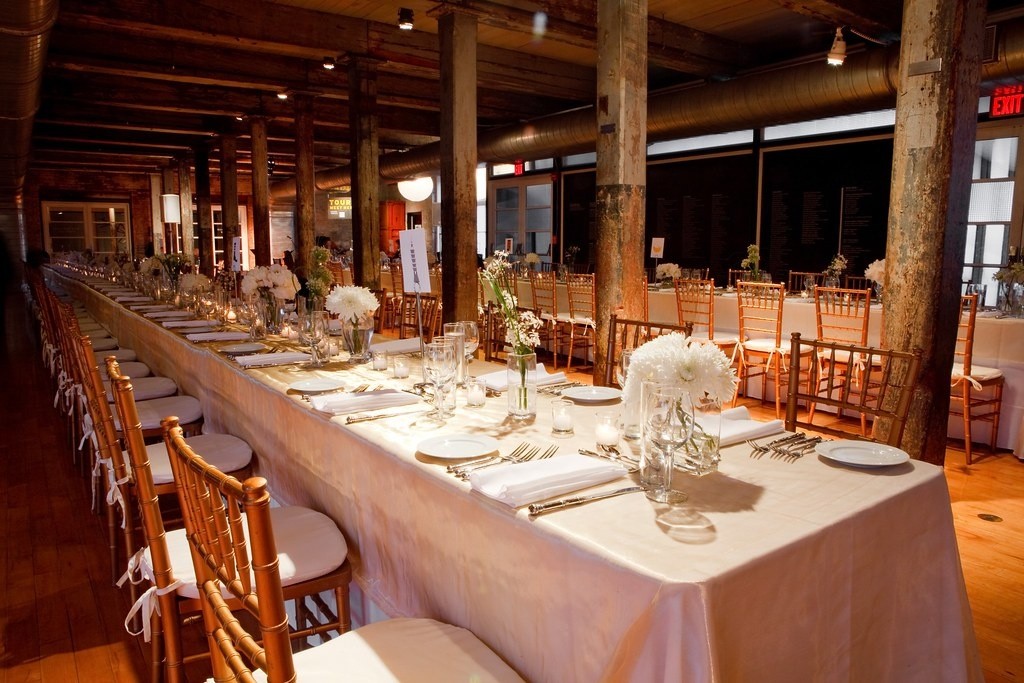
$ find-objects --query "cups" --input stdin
[966,282,987,311]
[639,380,673,490]
[674,266,772,294]
[249,299,268,340]
[311,312,330,367]
[432,322,467,411]
[806,275,816,300]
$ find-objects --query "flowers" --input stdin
[656,263,682,279]
[992,261,1024,287]
[620,332,740,437]
[863,258,887,283]
[824,253,848,278]
[524,252,540,269]
[741,244,761,281]
[97,249,388,321]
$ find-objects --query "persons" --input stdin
[318,235,331,250]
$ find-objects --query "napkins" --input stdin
[41,257,429,368]
[310,387,424,418]
[472,364,567,391]
[472,449,631,509]
[690,395,787,448]
[370,337,424,358]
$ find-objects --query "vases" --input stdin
[873,280,884,304]
[1008,281,1024,319]
[668,393,721,477]
[507,351,536,419]
[105,274,374,365]
[824,275,840,303]
[478,250,543,352]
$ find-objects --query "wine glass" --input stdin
[158,281,172,311]
[422,343,456,420]
[140,277,156,305]
[644,387,693,504]
[197,293,215,328]
[455,321,479,380]
[180,286,195,320]
[283,297,296,338]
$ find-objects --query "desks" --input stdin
[397,267,1024,463]
[23,251,990,633]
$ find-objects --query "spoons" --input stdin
[600,444,640,462]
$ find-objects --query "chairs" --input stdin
[105,357,354,683]
[163,415,531,682]
[327,256,1003,466]
[22,263,255,587]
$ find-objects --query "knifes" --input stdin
[529,487,644,514]
[347,407,425,424]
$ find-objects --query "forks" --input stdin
[302,384,384,401]
[447,441,560,479]
[579,449,638,473]
[745,431,833,458]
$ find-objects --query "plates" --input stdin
[561,385,623,403]
[418,433,498,458]
[108,281,217,332]
[289,377,346,391]
[218,343,264,352]
[815,440,910,469]
